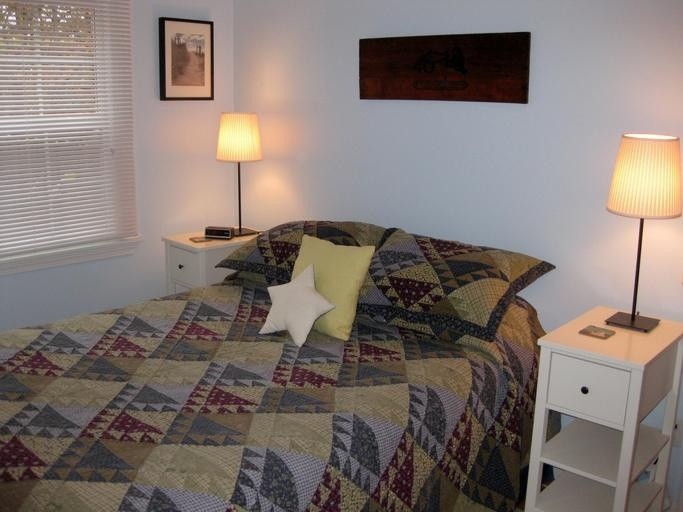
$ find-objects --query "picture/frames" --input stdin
[158,16,214,102]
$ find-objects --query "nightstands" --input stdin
[524,302,683,511]
[161,228,267,296]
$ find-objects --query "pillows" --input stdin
[356,225,554,338]
[214,216,388,278]
[291,233,375,342]
[257,263,334,346]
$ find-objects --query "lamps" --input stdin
[216,112,265,235]
[606,132,682,332]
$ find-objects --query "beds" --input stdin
[0,219,561,511]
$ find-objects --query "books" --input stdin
[579,326,615,339]
